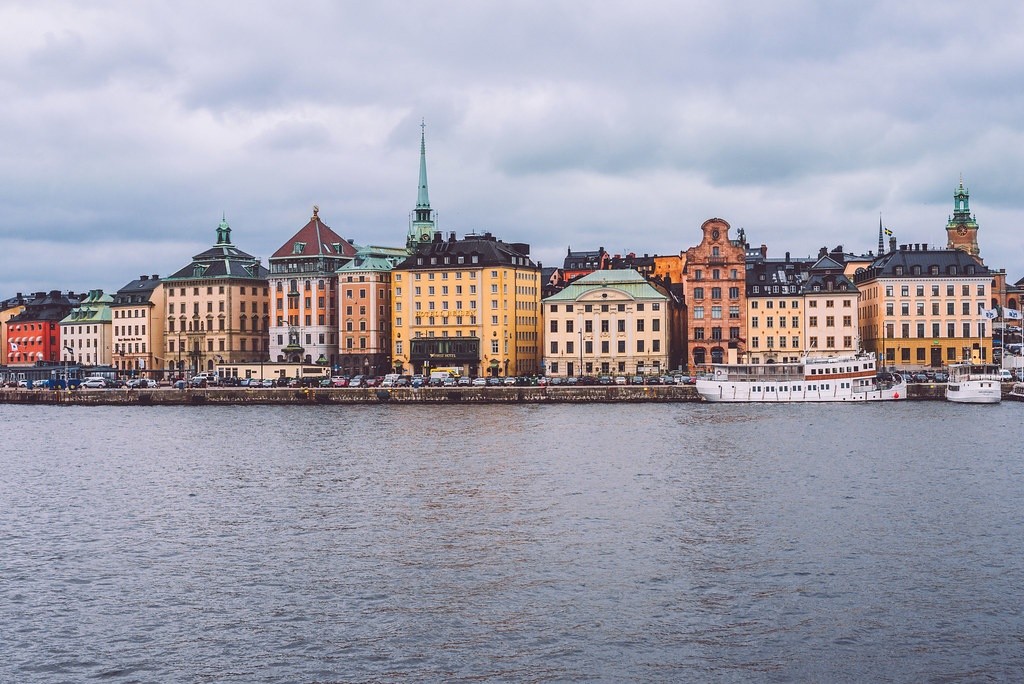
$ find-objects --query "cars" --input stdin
[992,321,1024,365]
[614,376,627,386]
[1001,370,1013,382]
[663,376,675,385]
[550,378,561,386]
[0,367,701,391]
[567,377,578,386]
[646,376,659,385]
[632,376,645,386]
[1015,371,1023,382]
[504,377,516,387]
[878,370,948,383]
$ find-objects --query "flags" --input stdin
[981,307,998,320]
[1003,307,1023,319]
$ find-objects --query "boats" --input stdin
[944,358,1002,404]
[695,339,907,404]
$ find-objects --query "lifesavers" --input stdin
[716,370,722,376]
